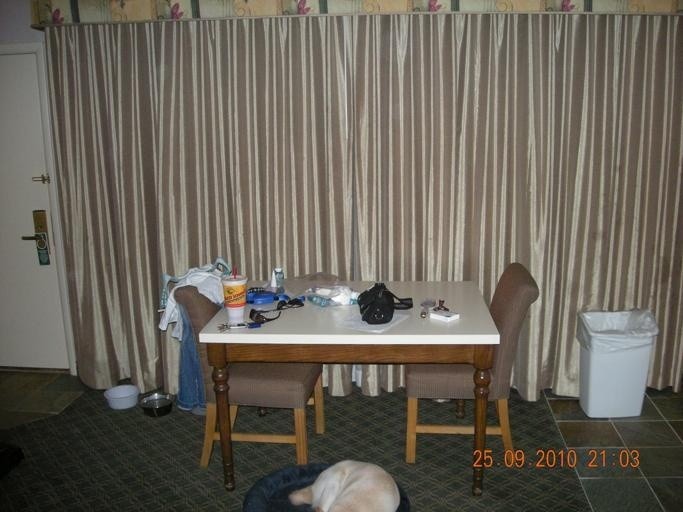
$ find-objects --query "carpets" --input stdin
[1,374,592,512]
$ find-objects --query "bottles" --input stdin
[274,267,286,294]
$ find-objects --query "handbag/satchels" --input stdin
[357,282,394,324]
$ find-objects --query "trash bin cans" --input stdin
[579,310,660,420]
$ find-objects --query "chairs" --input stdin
[405,264,538,464]
[176,285,325,468]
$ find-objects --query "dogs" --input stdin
[287,460,401,512]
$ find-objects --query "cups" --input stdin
[221,275,248,324]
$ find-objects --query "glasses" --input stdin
[249,298,304,324]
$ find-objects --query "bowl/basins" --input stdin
[103,384,139,410]
[140,393,173,417]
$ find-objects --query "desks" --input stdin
[198,281,501,498]
